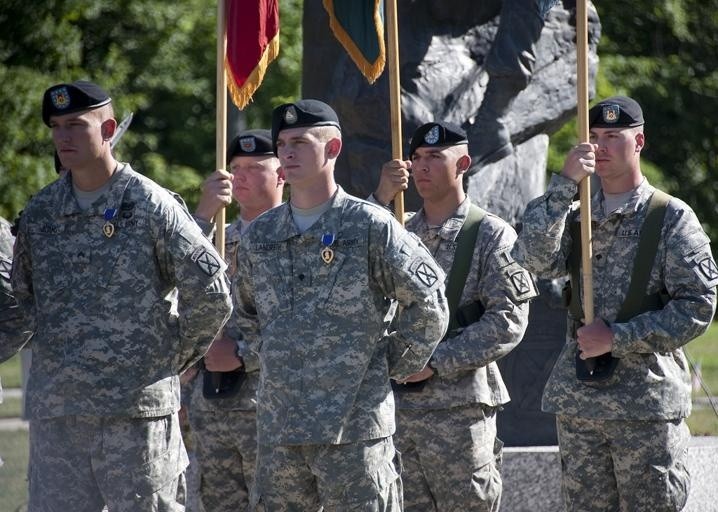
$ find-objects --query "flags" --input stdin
[223,0,279,112]
[303,0,395,84]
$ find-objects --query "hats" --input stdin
[408,124,467,159]
[42,81,111,127]
[589,95,644,129]
[270,100,341,158]
[226,129,272,165]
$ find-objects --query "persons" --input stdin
[230,98,451,512]
[10,80,234,512]
[0,215,35,471]
[510,96,718,512]
[364,121,541,512]
[181,129,285,512]
